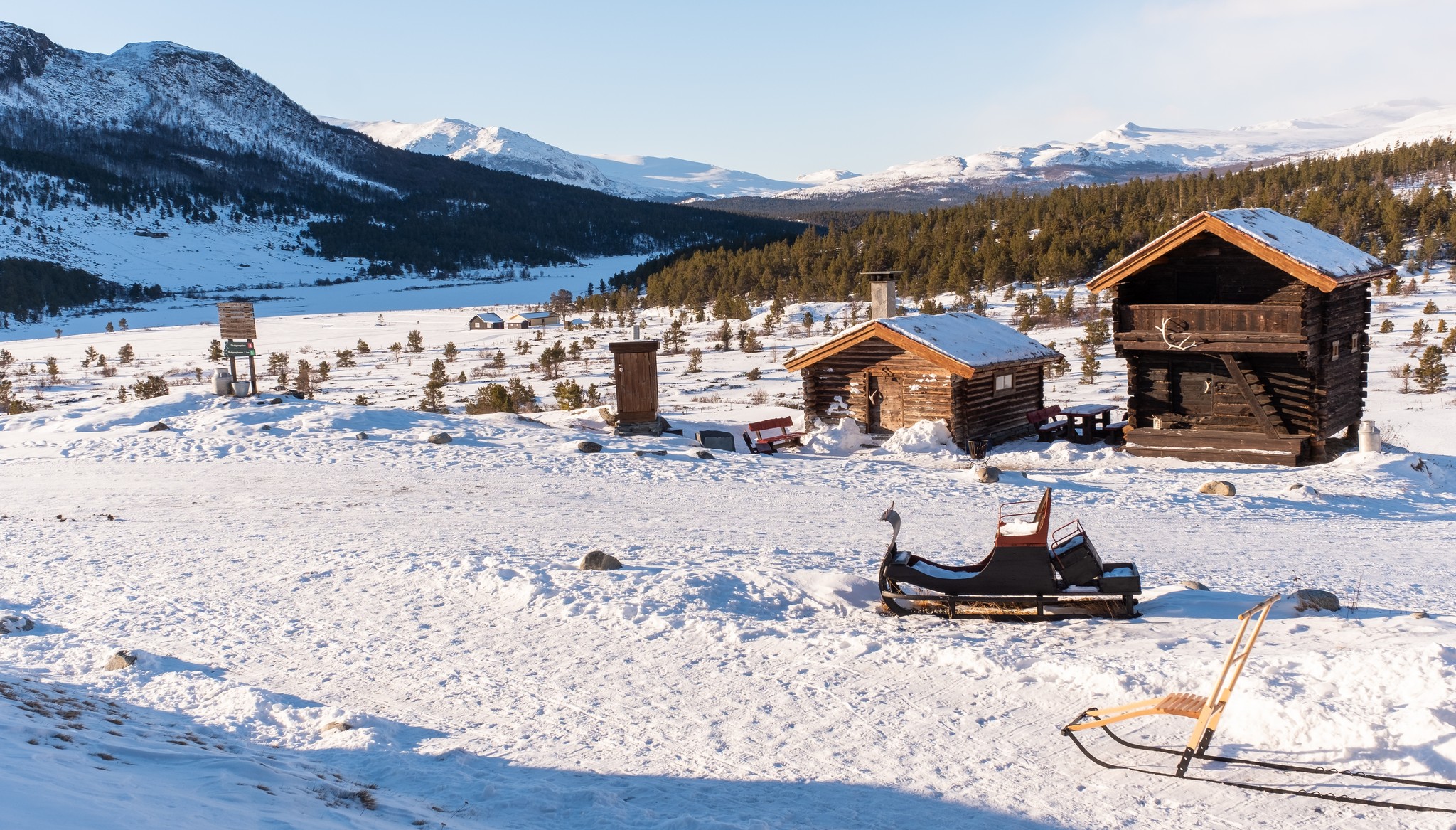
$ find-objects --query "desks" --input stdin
[1059,405,1119,444]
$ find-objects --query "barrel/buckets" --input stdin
[1358,421,1381,453]
[968,439,989,460]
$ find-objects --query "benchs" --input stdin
[748,416,808,453]
[1105,412,1128,444]
[1027,405,1078,442]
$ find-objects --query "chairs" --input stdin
[1156,592,1281,720]
[1051,517,1087,569]
[995,486,1052,547]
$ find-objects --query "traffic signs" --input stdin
[224,348,256,356]
[225,342,254,349]
